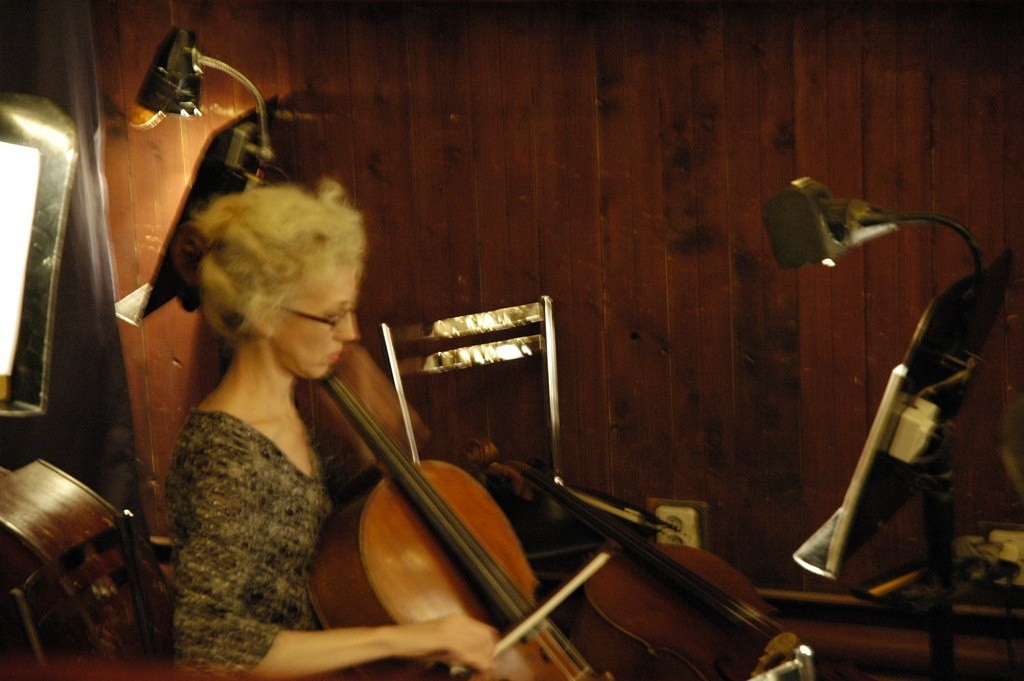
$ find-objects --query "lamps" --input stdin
[759,176,988,286]
[135,24,278,187]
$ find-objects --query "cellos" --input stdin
[296,365,629,680]
[457,432,892,681]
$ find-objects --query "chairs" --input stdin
[10,511,156,663]
[377,293,606,638]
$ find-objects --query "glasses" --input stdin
[279,301,372,332]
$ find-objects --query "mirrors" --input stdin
[0,92,80,419]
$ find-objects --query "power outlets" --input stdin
[646,496,712,554]
[975,519,1024,593]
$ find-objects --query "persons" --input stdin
[167,178,534,681]
